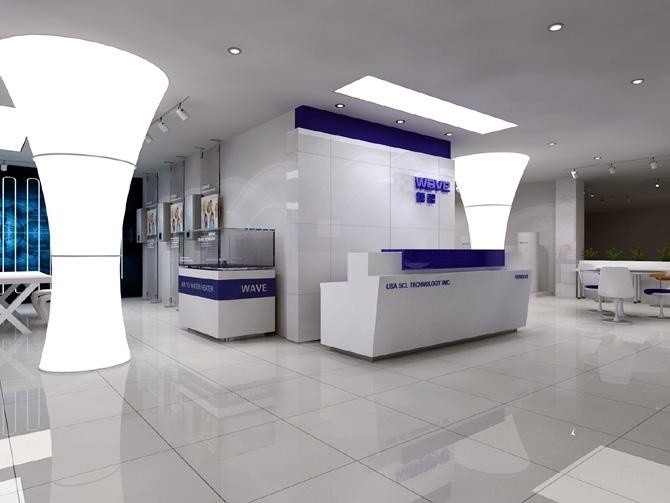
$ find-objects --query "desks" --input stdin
[0,271,52,335]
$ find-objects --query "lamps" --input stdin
[565,157,662,179]
[1,160,8,171]
[145,96,190,145]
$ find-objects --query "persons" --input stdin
[202,213,209,228]
[209,211,216,228]
[172,207,183,231]
[148,212,156,235]
[208,199,213,211]
[206,201,210,212]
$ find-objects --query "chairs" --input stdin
[31,288,51,324]
[578,262,670,324]
